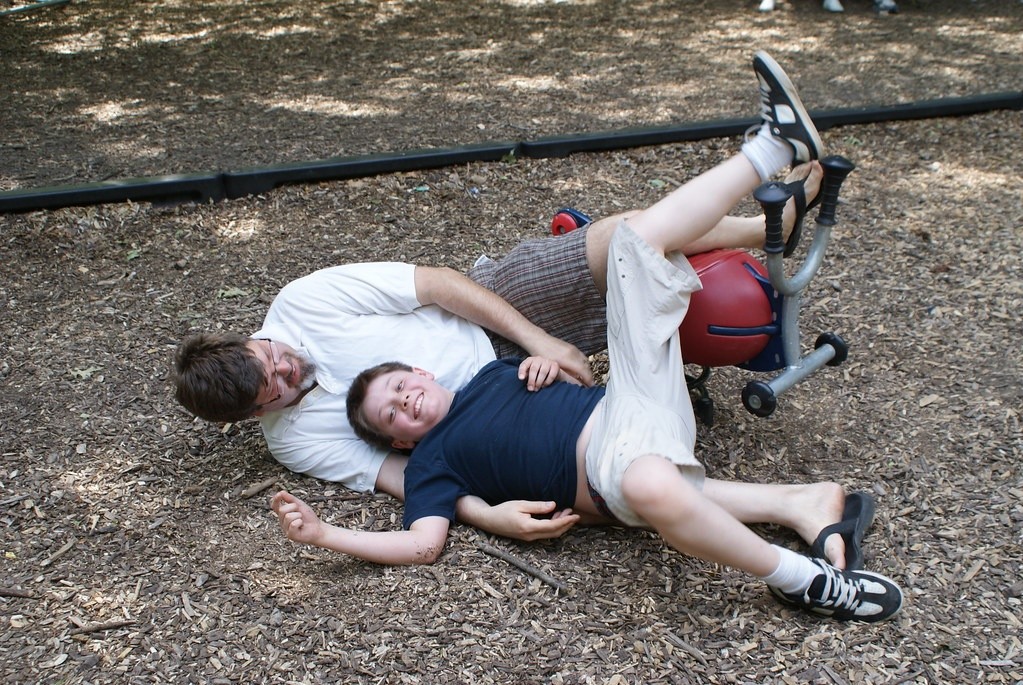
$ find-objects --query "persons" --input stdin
[265,54,909,626]
[169,157,846,576]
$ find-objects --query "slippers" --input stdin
[809,491,874,571]
[783,163,825,257]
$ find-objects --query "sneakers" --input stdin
[770,550,904,623]
[753,52,824,166]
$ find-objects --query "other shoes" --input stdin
[758,0,775,12]
[822,0,845,13]
[873,0,898,12]
